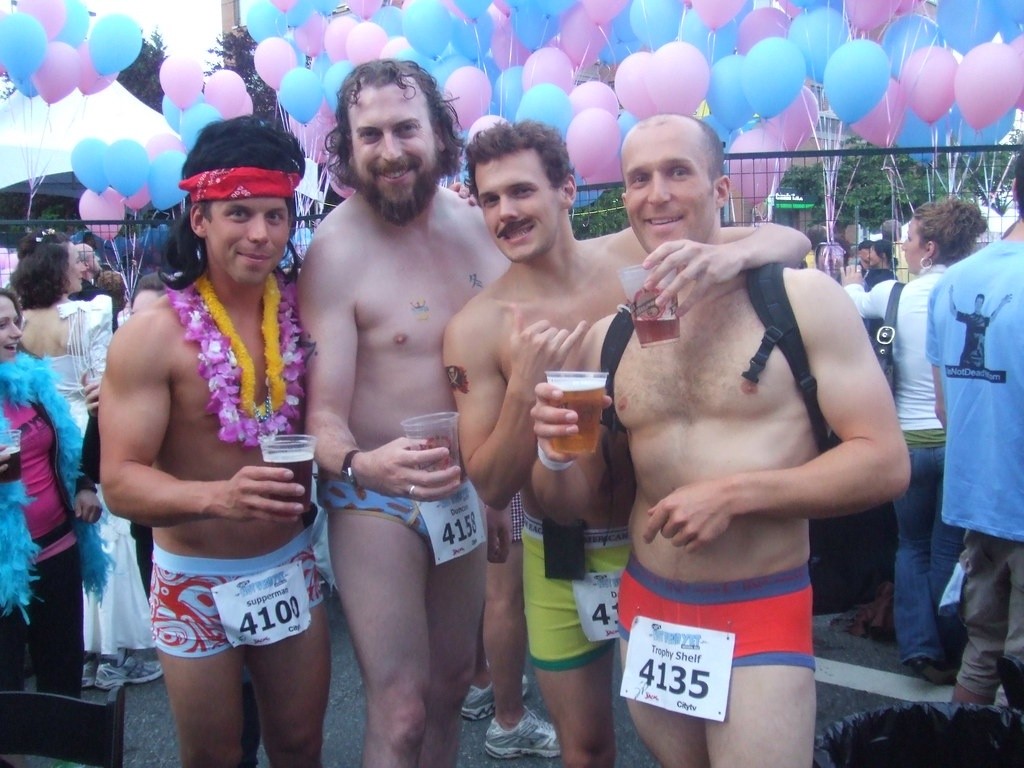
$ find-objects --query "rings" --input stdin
[856,269,862,273]
[408,485,415,498]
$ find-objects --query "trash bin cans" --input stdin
[811,700,1024,768]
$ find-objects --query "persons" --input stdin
[460,490,561,759]
[804,221,914,338]
[925,145,1024,713]
[296,58,513,768]
[530,112,912,768]
[79,272,262,768]
[0,229,164,699]
[97,113,476,768]
[440,117,812,768]
[839,195,987,685]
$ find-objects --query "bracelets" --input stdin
[535,441,576,472]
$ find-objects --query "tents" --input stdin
[0,73,184,200]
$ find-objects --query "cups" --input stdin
[0,429,23,483]
[542,370,608,454]
[258,434,316,513]
[88,377,105,391]
[400,411,460,491]
[616,259,680,348]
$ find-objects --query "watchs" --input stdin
[340,449,360,487]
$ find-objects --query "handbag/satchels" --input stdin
[874,283,905,407]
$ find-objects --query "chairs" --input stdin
[0,685,125,768]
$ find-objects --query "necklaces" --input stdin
[168,271,308,450]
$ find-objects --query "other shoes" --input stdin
[909,655,939,678]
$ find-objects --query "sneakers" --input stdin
[485,706,563,759]
[96,656,164,691]
[82,653,97,689]
[459,670,530,721]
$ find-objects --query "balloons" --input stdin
[0,0,142,106]
[159,54,253,152]
[71,134,190,239]
[246,0,1024,202]
